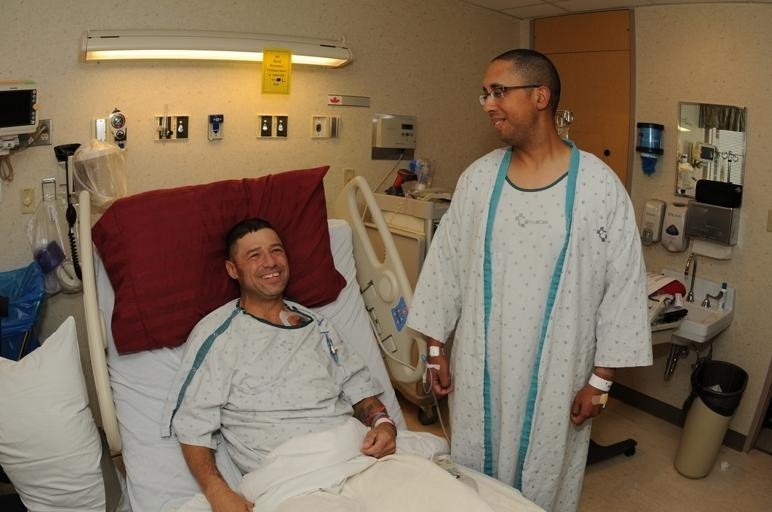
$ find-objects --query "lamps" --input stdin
[79,30,354,69]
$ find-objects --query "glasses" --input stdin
[479,84,542,107]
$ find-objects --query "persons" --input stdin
[404,48,653,512]
[161,217,399,512]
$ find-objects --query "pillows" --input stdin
[91,166,347,355]
[0,315,126,511]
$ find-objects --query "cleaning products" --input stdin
[716,280,728,312]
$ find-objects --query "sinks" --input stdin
[660,268,737,347]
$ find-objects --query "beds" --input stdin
[77,176,549,512]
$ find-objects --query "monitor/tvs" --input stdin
[0,80,39,136]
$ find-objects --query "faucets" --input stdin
[683,251,697,302]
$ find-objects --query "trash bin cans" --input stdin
[673,359,748,482]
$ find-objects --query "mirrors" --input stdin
[673,102,747,199]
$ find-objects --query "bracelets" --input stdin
[589,372,614,393]
[424,344,447,358]
[367,407,398,437]
